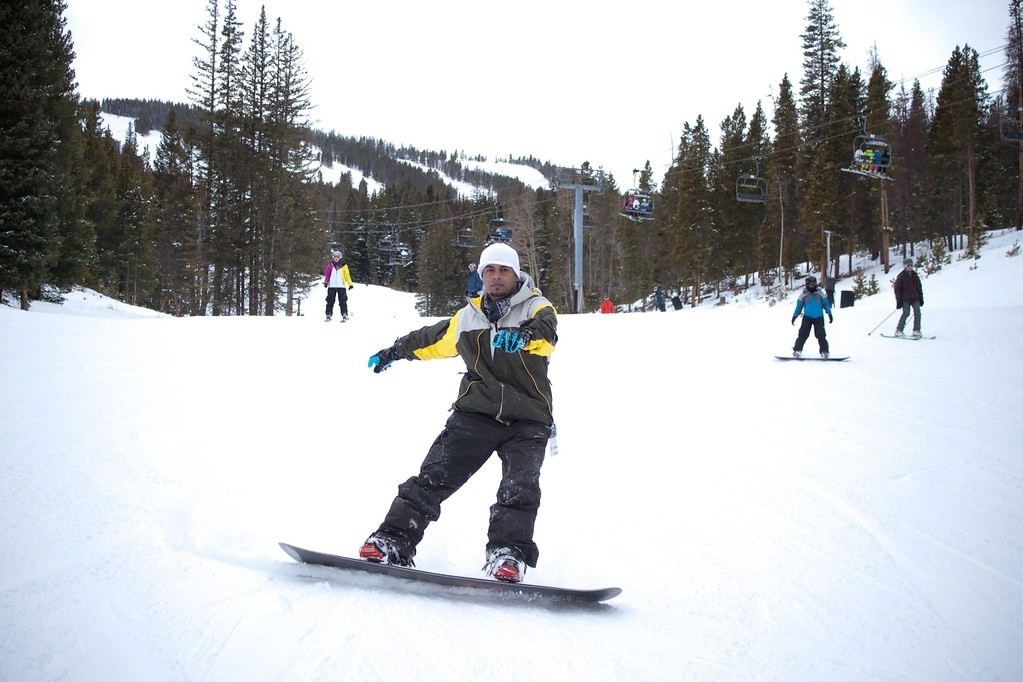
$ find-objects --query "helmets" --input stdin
[468,263,477,272]
[805,277,818,292]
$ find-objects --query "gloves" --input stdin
[829,315,834,324]
[920,301,924,306]
[324,283,328,288]
[491,329,532,353]
[897,304,902,309]
[348,286,353,291]
[791,317,796,326]
[368,346,398,373]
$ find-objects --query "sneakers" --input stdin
[360,534,416,565]
[488,549,527,581]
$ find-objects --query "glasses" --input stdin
[906,264,913,267]
[334,256,340,258]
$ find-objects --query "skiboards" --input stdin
[880,333,936,341]
[324,320,347,323]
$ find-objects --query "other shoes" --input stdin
[793,351,803,358]
[913,331,922,337]
[820,352,830,358]
[895,329,905,336]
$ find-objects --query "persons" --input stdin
[464,263,483,299]
[359,244,559,582]
[324,250,353,322]
[892,259,924,338]
[600,297,614,314]
[791,276,833,358]
[484,226,513,245]
[623,197,653,219]
[854,147,891,175]
[653,286,665,313]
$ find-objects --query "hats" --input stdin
[477,243,521,280]
[333,251,342,256]
[903,258,914,267]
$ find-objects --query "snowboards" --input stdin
[275,539,623,602]
[775,354,851,363]
